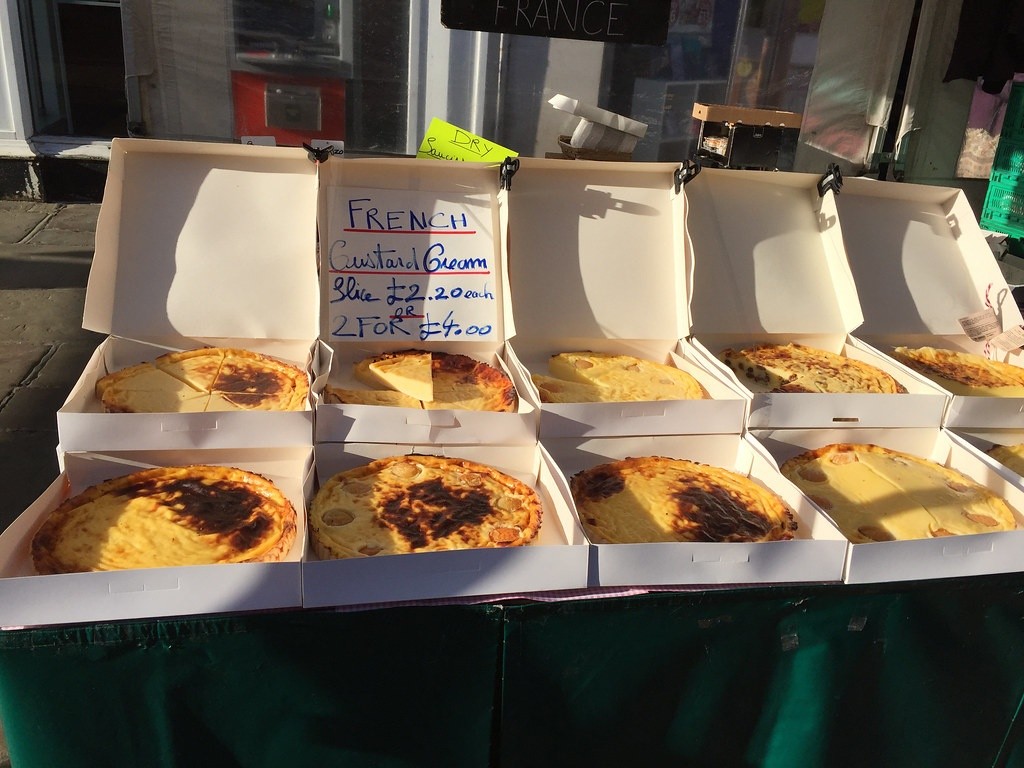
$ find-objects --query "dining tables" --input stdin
[0,574,1024,768]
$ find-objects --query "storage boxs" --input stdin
[0,137,1024,631]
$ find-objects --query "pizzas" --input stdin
[323,348,516,413]
[311,456,543,558]
[30,462,296,573]
[778,442,1017,544]
[890,344,1024,398]
[531,352,703,403]
[96,347,310,412]
[715,341,907,394]
[568,454,796,543]
[984,443,1024,478]
